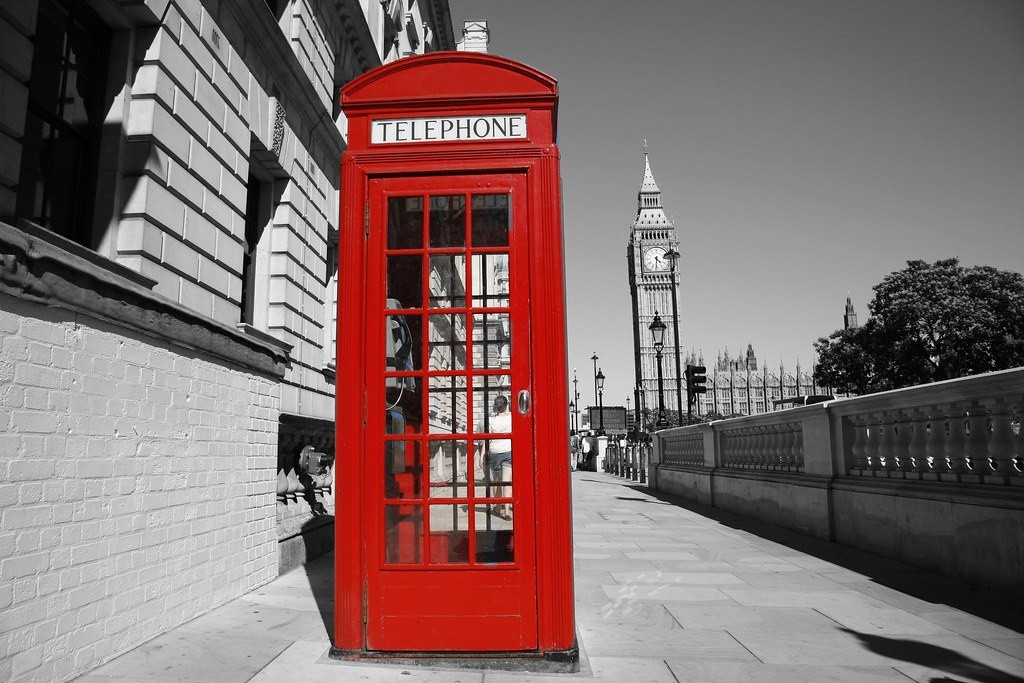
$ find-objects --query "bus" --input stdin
[772,395,835,411]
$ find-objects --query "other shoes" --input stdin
[493,507,501,516]
[504,511,513,521]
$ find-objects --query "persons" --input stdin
[569,429,578,471]
[479,395,514,521]
[581,433,594,470]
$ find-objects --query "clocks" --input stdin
[644,247,669,271]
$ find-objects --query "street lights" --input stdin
[663,242,684,427]
[626,396,631,431]
[569,399,575,436]
[591,352,599,406]
[595,368,607,437]
[573,370,579,435]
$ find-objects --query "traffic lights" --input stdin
[687,364,707,394]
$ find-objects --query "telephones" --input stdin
[386,297,416,392]
